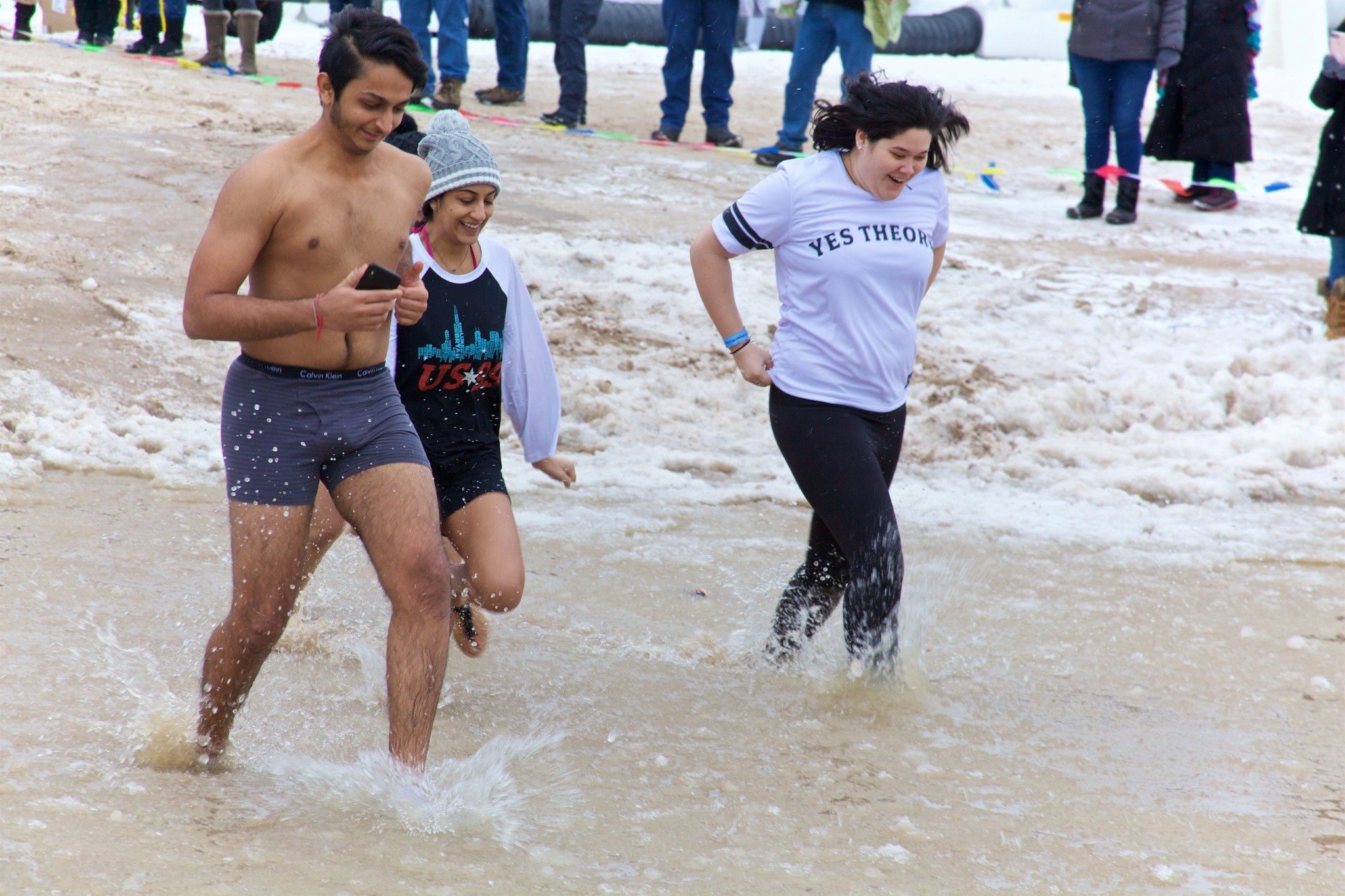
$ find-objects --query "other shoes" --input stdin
[94,33,112,46]
[540,110,585,128]
[76,30,94,45]
[407,87,427,108]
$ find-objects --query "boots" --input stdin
[431,77,463,106]
[1316,275,1345,339]
[13,3,35,42]
[1067,171,1105,219]
[126,16,163,54]
[152,18,182,55]
[1106,175,1141,224]
[195,9,230,65]
[233,9,263,74]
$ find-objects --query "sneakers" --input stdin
[651,126,679,142]
[1176,184,1208,203]
[475,84,524,104]
[705,125,740,146]
[1193,187,1236,210]
[756,142,803,167]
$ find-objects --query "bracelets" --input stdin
[312,292,323,342]
[730,340,750,354]
[724,329,747,348]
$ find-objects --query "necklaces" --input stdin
[428,237,469,272]
[839,153,855,185]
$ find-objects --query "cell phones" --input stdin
[353,261,401,290]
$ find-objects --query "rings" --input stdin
[756,382,761,385]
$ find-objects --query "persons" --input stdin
[689,74,970,673]
[1297,24,1345,340]
[1063,0,1186,223]
[399,0,470,111]
[1141,0,1262,210]
[757,0,910,168]
[328,0,374,21]
[650,0,742,147]
[13,0,188,56]
[540,0,602,126]
[473,0,530,104]
[193,0,263,75]
[301,108,575,657]
[184,4,450,767]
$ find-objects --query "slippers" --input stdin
[452,603,488,658]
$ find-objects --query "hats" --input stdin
[418,109,502,205]
